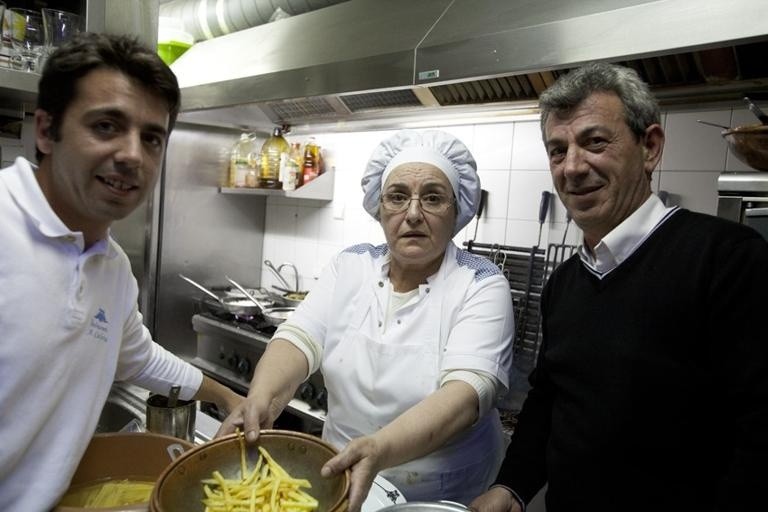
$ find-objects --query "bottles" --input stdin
[228,126,319,191]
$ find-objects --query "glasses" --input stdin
[378,191,457,215]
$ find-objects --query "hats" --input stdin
[361,128,482,238]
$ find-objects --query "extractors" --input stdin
[167,1,768,129]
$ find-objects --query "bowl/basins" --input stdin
[720,126,766,173]
[148,429,352,511]
[55,432,197,511]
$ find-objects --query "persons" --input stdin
[212,128,516,512]
[468,62,767,511]
[1,32,247,512]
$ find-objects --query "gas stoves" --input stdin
[193,288,328,427]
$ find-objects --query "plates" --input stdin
[361,472,407,512]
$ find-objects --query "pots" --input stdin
[177,259,308,327]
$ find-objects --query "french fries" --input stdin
[200,426,319,512]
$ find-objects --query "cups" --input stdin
[144,394,197,442]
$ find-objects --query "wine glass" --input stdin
[7,5,84,77]
